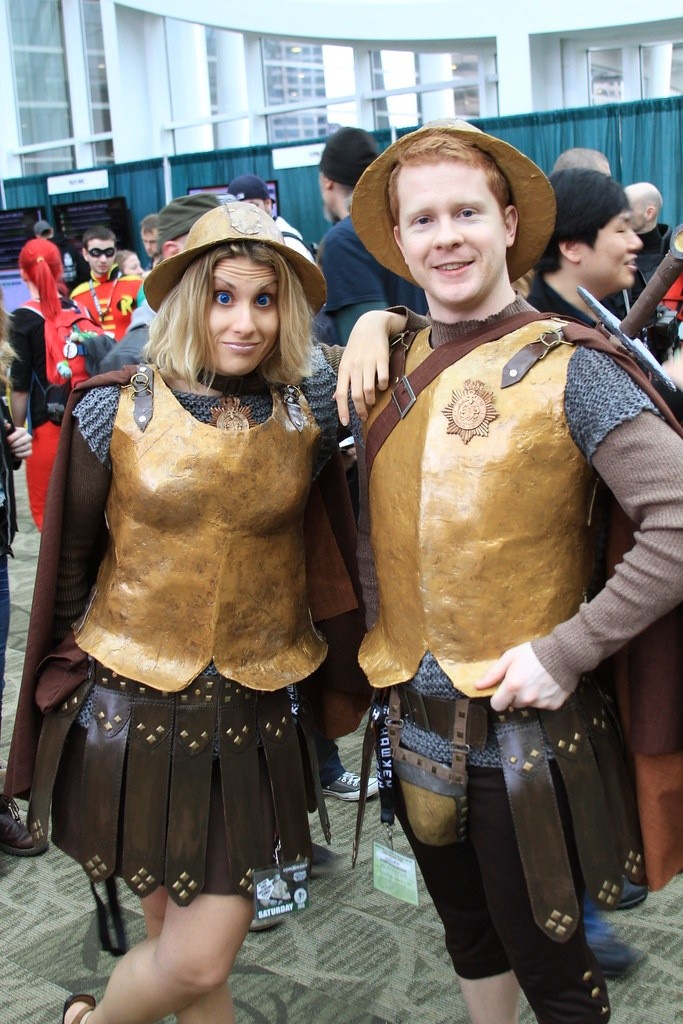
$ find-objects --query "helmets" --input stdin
[143,202,327,317]
[349,115,557,286]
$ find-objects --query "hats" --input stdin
[157,192,223,253]
[227,174,276,203]
[319,126,378,186]
[33,221,51,236]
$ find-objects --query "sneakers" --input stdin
[323,771,379,800]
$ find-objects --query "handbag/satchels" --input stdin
[391,747,468,846]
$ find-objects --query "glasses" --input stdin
[86,247,116,257]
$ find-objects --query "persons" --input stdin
[6,201,427,1024]
[0,126,428,928]
[526,147,683,978]
[355,121,683,1024]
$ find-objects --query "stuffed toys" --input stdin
[56,361,73,378]
[70,331,97,342]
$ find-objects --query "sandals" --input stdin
[62,994,96,1024]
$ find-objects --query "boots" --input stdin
[0,758,49,856]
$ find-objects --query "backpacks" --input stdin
[18,300,104,389]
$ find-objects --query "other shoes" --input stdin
[616,878,648,909]
[588,938,634,978]
